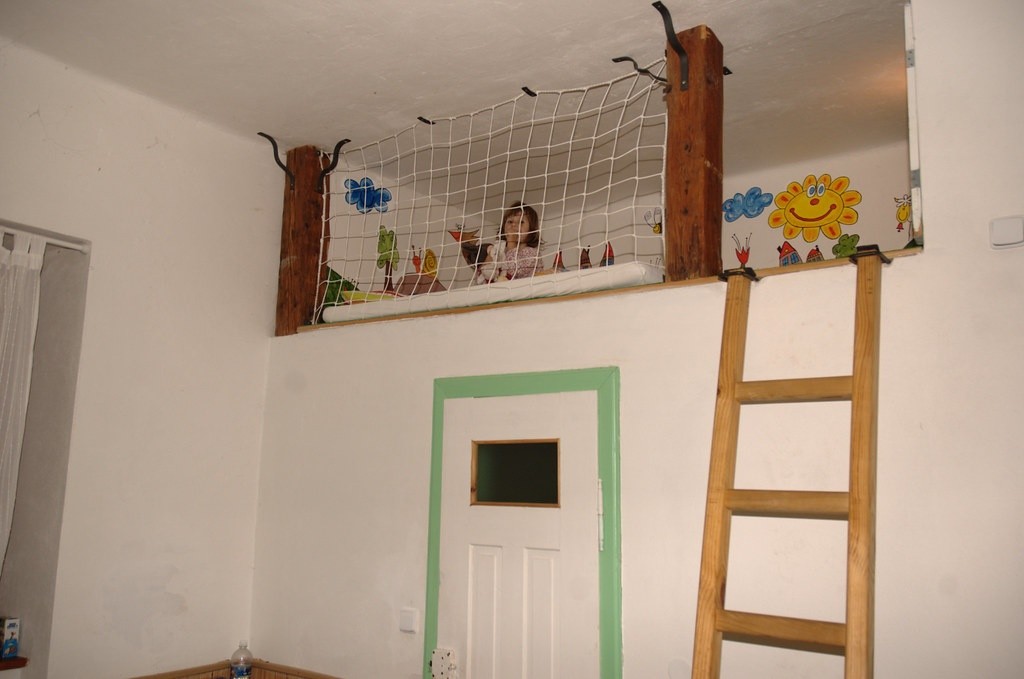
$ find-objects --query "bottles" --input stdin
[230,640,252,679]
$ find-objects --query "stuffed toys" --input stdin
[469,243,508,282]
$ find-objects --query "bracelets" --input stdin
[508,240,518,243]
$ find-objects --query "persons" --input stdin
[476,201,544,283]
